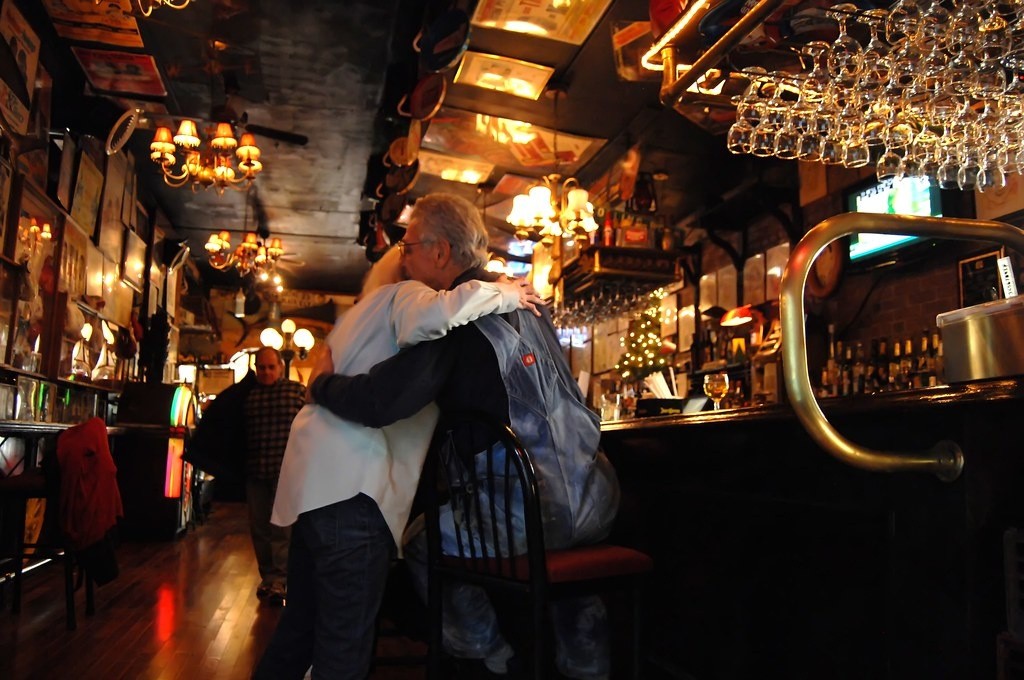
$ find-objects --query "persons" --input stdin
[249,246,546,680]
[237,346,306,600]
[305,194,620,679]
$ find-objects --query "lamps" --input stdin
[150,50,262,196]
[203,223,309,279]
[505,83,599,246]
[720,307,766,327]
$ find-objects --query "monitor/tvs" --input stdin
[841,168,947,267]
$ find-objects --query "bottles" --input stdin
[820,326,939,396]
[689,329,733,368]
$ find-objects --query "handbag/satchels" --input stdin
[427,445,530,560]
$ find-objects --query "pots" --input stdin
[937,295,1023,383]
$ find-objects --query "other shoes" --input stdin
[256,580,270,597]
[269,580,285,600]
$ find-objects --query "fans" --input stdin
[141,86,310,147]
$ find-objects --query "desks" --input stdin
[0,418,127,616]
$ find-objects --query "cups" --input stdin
[601,394,620,422]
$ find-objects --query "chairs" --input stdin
[0,416,115,631]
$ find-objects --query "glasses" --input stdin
[398,240,425,256]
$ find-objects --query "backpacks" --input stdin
[474,291,620,551]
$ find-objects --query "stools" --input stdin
[368,414,653,680]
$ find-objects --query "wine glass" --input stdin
[728,0,1024,194]
[704,374,729,411]
